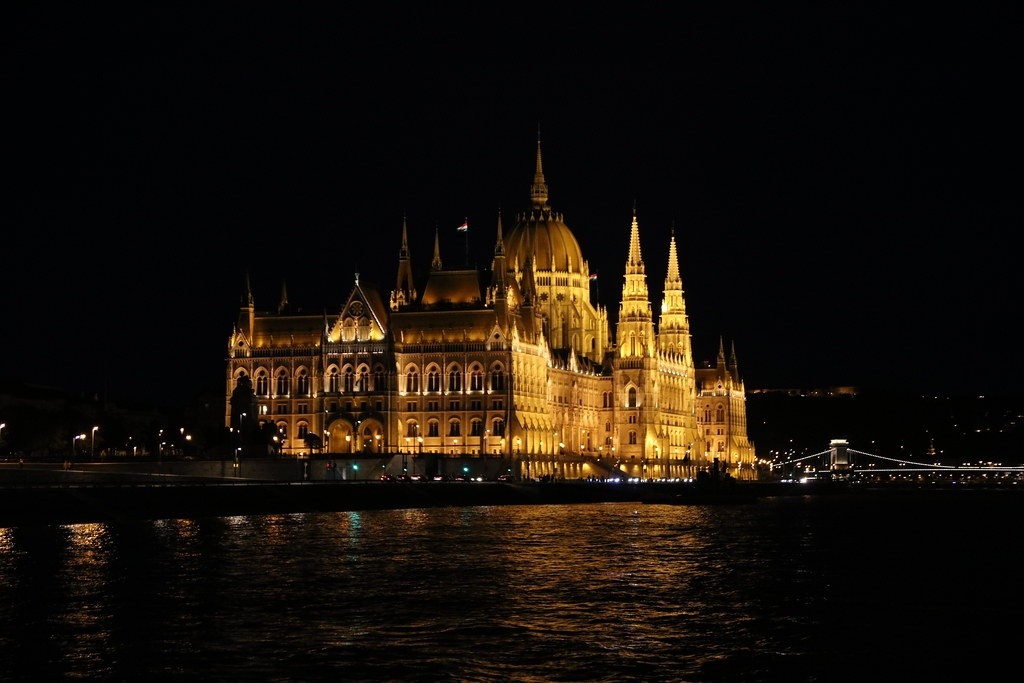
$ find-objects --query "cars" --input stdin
[379,472,488,482]
[497,474,511,483]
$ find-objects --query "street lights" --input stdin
[412,424,419,475]
[485,430,489,454]
[72,435,80,457]
[608,436,612,454]
[235,446,242,477]
[91,426,99,457]
